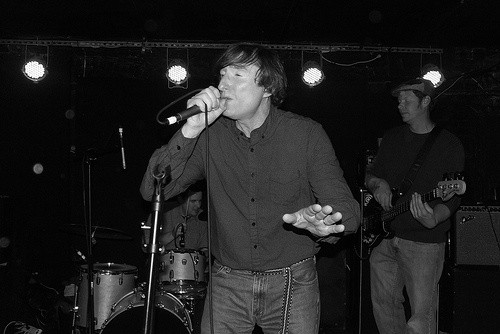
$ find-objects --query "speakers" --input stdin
[454,206,500,267]
[439,262,500,334]
[316,247,361,333]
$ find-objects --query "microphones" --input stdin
[119,128,127,170]
[166,97,221,125]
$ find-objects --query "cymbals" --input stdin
[67,223,130,241]
[198,208,208,221]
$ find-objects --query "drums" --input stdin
[99,287,195,334]
[72,261,139,332]
[152,246,207,303]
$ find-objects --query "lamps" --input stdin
[166,48,190,90]
[22,43,49,82]
[300,49,326,88]
[419,53,445,88]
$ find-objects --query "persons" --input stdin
[140,189,208,253]
[361,77,470,334]
[140,42,360,334]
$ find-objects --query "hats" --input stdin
[391,79,434,98]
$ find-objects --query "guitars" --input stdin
[353,176,469,259]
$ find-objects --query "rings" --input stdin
[323,221,327,225]
